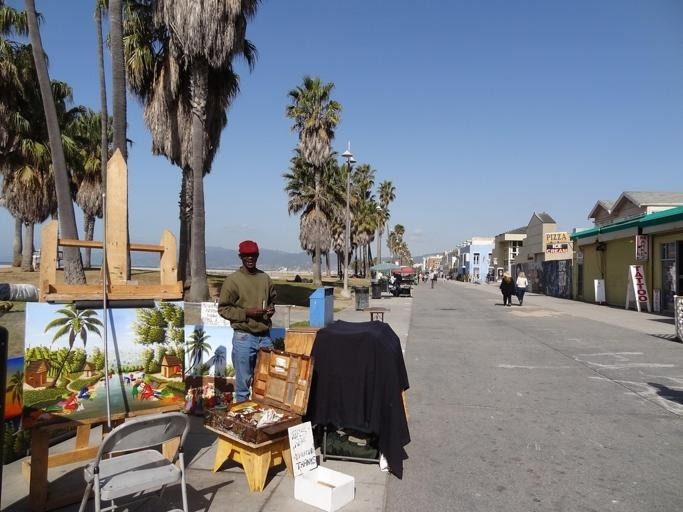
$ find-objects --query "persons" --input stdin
[416,268,494,288]
[516,271,528,305]
[285,275,313,283]
[500,270,515,307]
[393,272,402,290]
[217,240,278,404]
[358,268,362,278]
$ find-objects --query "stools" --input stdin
[213,433,294,492]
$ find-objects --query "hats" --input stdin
[238,241,259,255]
[502,271,512,278]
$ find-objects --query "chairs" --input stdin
[78,411,191,511]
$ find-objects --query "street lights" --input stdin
[462,265,465,282]
[343,139,357,291]
[376,205,382,264]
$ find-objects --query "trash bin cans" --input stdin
[355,287,369,310]
[309,287,333,327]
[372,282,382,299]
[673,295,683,342]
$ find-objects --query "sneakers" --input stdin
[503,303,512,308]
[519,299,523,306]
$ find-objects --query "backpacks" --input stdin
[433,274,437,281]
